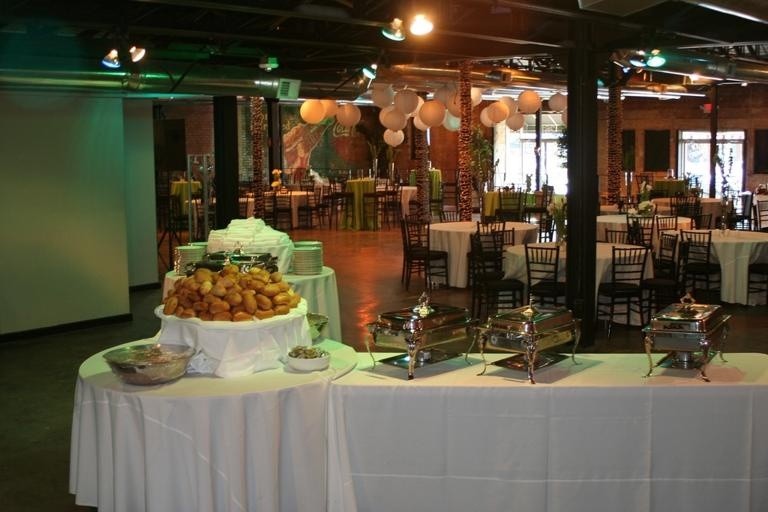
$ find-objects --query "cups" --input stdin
[357,169,364,181]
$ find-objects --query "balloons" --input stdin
[300,83,570,148]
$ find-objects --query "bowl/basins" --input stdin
[287,350,330,370]
[305,313,328,340]
[102,343,196,385]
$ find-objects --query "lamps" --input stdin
[381,8,438,42]
[360,58,379,80]
[629,34,668,70]
[99,27,146,69]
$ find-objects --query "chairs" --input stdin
[160,159,476,245]
[400,171,767,339]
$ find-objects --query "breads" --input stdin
[161,264,300,321]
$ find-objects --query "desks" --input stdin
[162,265,338,346]
[70,332,363,510]
[328,348,768,512]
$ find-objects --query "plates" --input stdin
[172,240,207,275]
[292,239,323,274]
